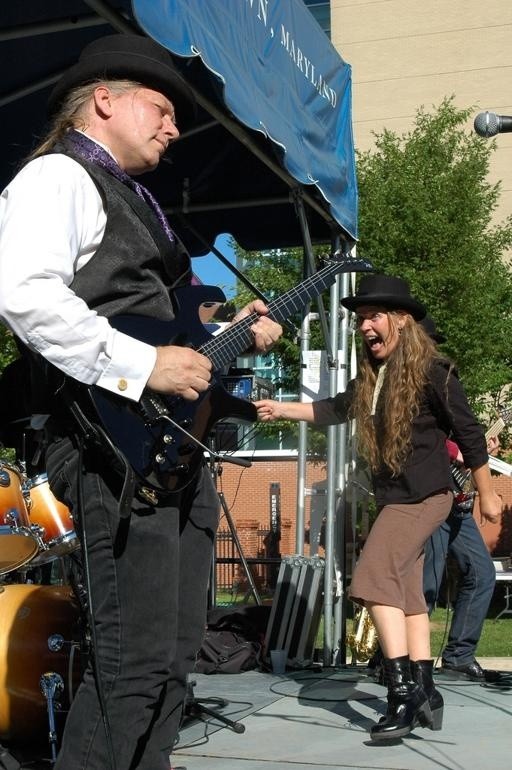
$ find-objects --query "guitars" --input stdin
[63,250,377,508]
[450,412,512,513]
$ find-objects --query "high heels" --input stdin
[370,655,444,740]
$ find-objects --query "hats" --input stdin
[47,33,196,127]
[341,275,427,321]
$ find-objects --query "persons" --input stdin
[370,435,502,681]
[0,34,284,770]
[252,275,502,740]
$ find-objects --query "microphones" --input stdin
[474,110,512,139]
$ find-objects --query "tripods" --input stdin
[177,680,246,734]
[208,440,263,610]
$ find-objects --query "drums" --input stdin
[0,458,47,574]
[23,472,82,566]
[0,579,89,760]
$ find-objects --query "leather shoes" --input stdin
[442,660,502,681]
